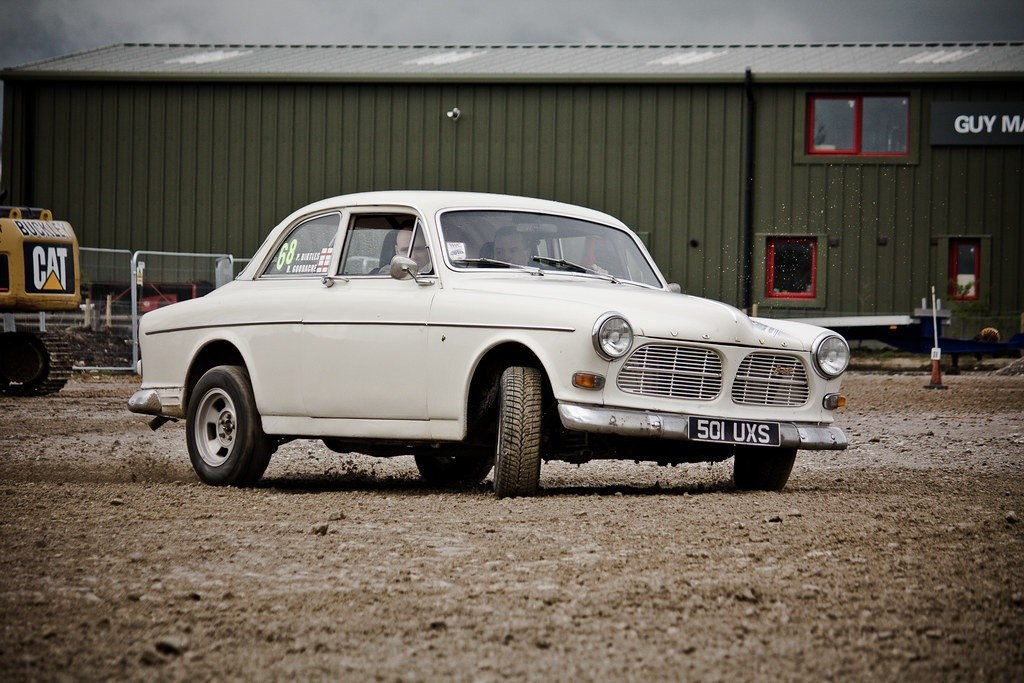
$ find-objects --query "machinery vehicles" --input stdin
[0,202,82,398]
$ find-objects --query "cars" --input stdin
[128,189,853,500]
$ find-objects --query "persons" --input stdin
[493,227,608,274]
[378,224,432,275]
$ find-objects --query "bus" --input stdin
[87,280,213,317]
